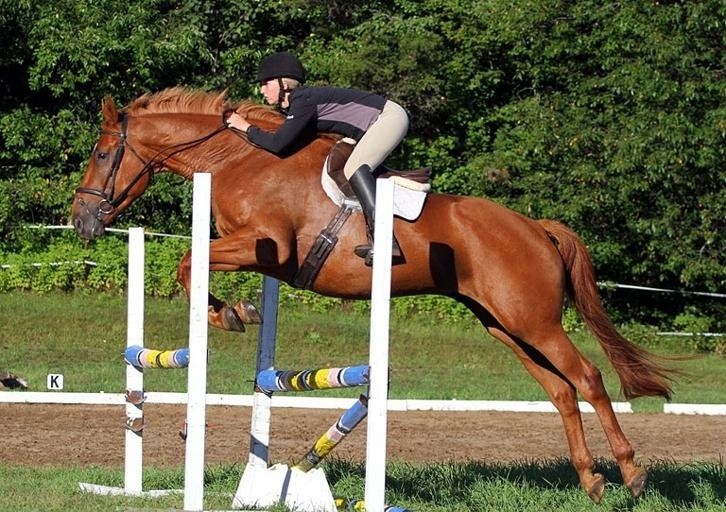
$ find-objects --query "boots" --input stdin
[346,164,403,262]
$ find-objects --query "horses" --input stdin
[71,87,709,504]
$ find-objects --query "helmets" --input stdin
[249,51,309,87]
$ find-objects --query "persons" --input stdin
[223,50,410,259]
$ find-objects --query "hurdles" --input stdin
[79,172,403,511]
